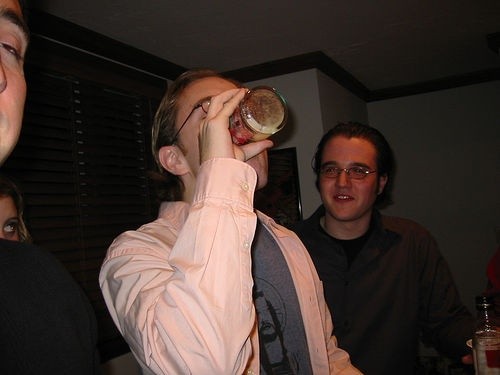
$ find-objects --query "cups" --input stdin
[228,85,288,146]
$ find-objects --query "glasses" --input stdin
[316,165,377,179]
[171,99,212,146]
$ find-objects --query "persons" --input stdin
[0,176,30,243]
[99,67,365,375]
[295,122,483,375]
[0,0,104,375]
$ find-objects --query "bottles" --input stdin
[473,294,500,375]
